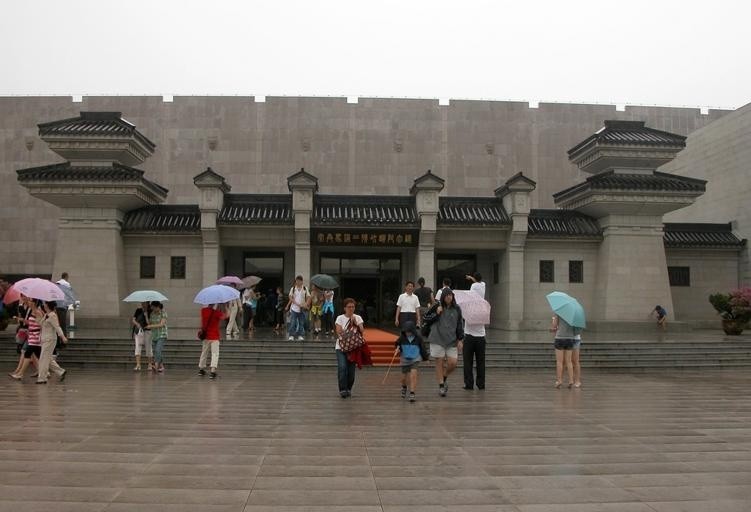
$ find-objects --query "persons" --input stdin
[2,272,74,385]
[394,272,491,403]
[272,276,334,343]
[562,331,589,388]
[222,283,262,336]
[130,302,153,372]
[197,303,231,379]
[144,301,168,373]
[648,304,668,332]
[334,298,363,399]
[550,315,574,389]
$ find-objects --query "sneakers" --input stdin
[444,381,449,391]
[463,386,474,390]
[58,369,67,382]
[275,327,284,330]
[249,327,256,330]
[134,365,164,372]
[408,392,416,402]
[10,370,51,384]
[209,372,217,379]
[401,386,407,398]
[341,390,351,397]
[198,370,206,375]
[439,388,446,396]
[289,336,295,341]
[226,330,239,335]
[298,336,304,341]
[275,327,335,335]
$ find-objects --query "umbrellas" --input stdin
[312,273,339,290]
[237,276,263,291]
[215,275,244,287]
[545,291,587,329]
[122,290,168,302]
[193,284,241,306]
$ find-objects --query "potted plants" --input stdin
[708,285,751,335]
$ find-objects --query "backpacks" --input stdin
[421,302,442,337]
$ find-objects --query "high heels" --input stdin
[554,381,582,388]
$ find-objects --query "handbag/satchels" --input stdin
[136,329,146,347]
[197,327,207,340]
[338,326,364,352]
